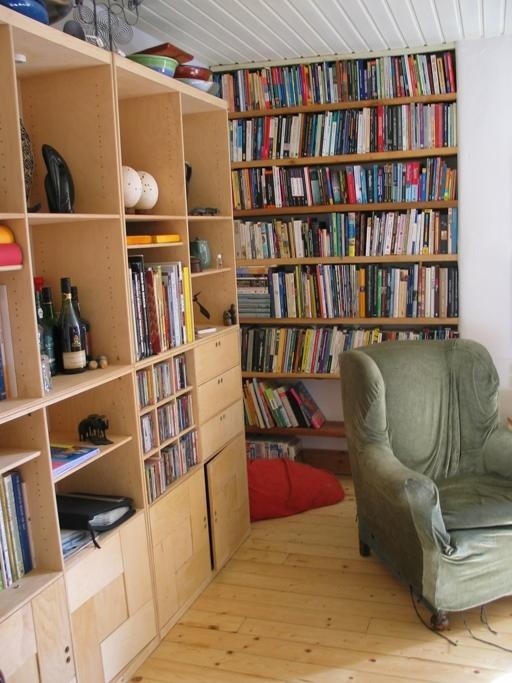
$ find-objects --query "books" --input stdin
[59,529,100,563]
[241,376,326,466]
[232,155,456,210]
[126,251,199,505]
[241,325,456,375]
[0,281,20,401]
[0,468,36,590]
[212,52,455,112]
[228,101,456,162]
[236,261,456,318]
[234,207,457,260]
[51,441,100,480]
[55,490,136,534]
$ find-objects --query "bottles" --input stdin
[33,275,91,374]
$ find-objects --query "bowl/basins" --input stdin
[125,54,179,78]
[174,64,213,91]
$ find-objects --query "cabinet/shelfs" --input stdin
[204,41,459,443]
[0,2,253,683]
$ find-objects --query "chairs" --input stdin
[336,336,512,630]
